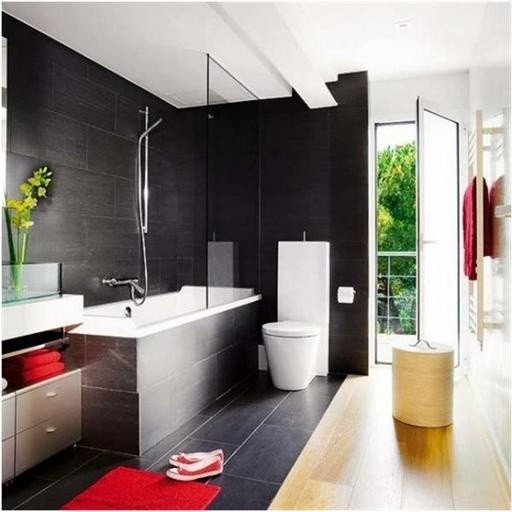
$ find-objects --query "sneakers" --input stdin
[168,448,224,466]
[167,456,223,481]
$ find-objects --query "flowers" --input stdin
[3,166,54,283]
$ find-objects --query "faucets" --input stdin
[114,279,145,295]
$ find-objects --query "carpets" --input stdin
[62,466,223,510]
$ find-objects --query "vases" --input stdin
[3,207,32,296]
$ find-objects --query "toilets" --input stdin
[262,320,322,390]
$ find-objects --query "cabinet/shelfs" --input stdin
[2,368,83,485]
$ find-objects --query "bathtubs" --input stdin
[73,285,262,339]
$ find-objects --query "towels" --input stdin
[464,176,494,281]
[15,350,65,382]
